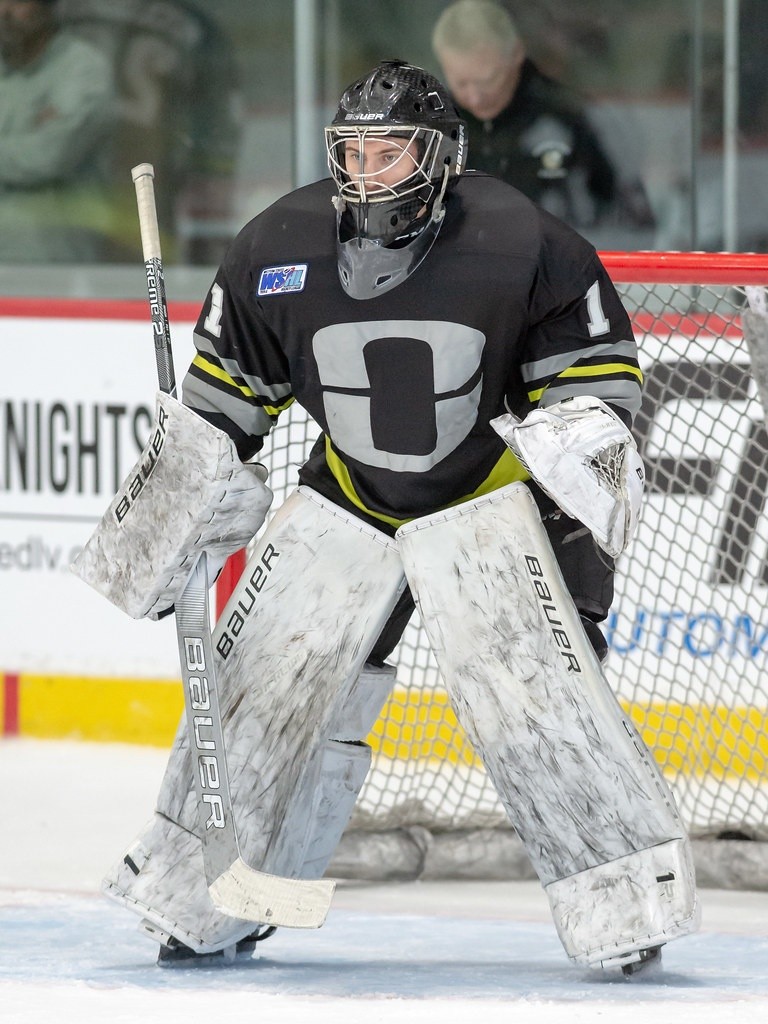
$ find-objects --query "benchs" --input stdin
[160,83,768,267]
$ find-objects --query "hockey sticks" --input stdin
[130,160,339,930]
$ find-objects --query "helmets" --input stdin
[324,58,467,300]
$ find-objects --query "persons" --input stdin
[433,0,614,227]
[53,0,242,266]
[71,58,698,972]
[0,0,113,268]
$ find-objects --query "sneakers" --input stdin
[620,942,665,982]
[157,927,259,967]
